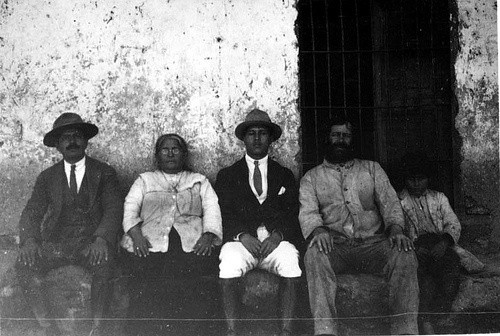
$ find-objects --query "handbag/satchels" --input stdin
[418,232,460,298]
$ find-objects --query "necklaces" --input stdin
[159,167,185,193]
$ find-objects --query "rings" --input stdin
[198,243,201,246]
[136,246,139,251]
[206,247,208,250]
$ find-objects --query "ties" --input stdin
[254,161,262,196]
[70,165,77,205]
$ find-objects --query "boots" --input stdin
[278,277,301,336]
[218,278,242,336]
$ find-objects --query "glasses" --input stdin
[155,149,180,156]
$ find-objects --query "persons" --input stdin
[298,115,419,335]
[215,108,303,336]
[14,113,123,336]
[399,167,461,334]
[119,134,228,336]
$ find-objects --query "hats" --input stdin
[235,109,282,142]
[398,152,437,176]
[44,113,98,147]
